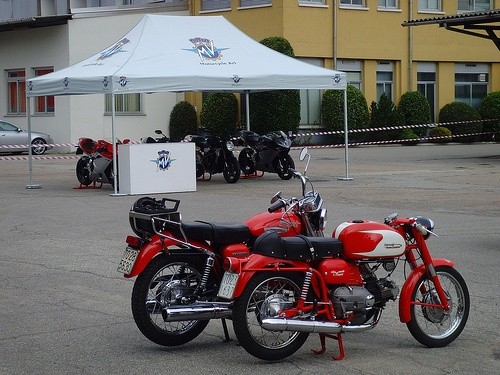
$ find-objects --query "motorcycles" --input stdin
[75,135,130,192]
[238,127,295,180]
[216,211,470,362]
[117,145,328,348]
[188,129,240,184]
[140,129,205,180]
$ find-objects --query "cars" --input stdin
[0,120,54,155]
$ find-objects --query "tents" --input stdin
[24,14,353,197]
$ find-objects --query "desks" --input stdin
[116,141,197,197]
